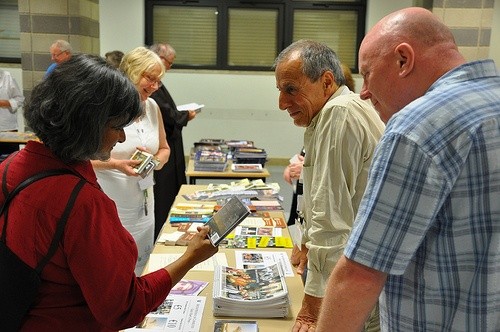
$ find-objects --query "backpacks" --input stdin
[0,144,86,331]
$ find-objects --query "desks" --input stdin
[120,184,305,332]
[0,131,40,162]
[186,147,271,185]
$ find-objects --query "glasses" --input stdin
[50,50,66,57]
[143,75,162,87]
[161,56,172,66]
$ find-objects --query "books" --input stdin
[175,101,205,116]
[122,139,295,332]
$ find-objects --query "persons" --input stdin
[0,40,126,155]
[89,48,171,277]
[273,39,386,332]
[149,44,197,243]
[315,7,500,332]
[0,53,219,332]
